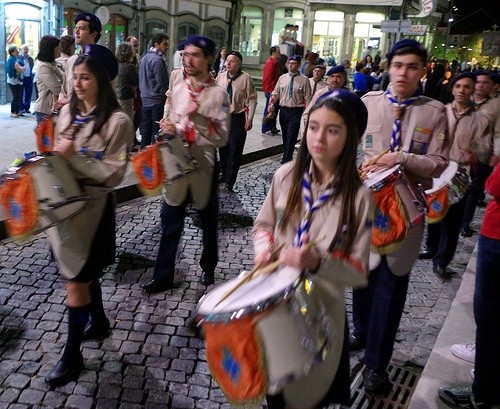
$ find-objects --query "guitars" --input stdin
[362,164,431,252]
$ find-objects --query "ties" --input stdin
[312,78,322,96]
[284,171,336,298]
[449,102,472,149]
[384,88,422,152]
[64,111,97,140]
[473,97,490,110]
[288,73,300,97]
[184,76,212,142]
[226,71,242,104]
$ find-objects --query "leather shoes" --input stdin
[141,277,172,294]
[45,347,83,387]
[349,191,474,404]
[80,318,111,341]
[200,269,214,285]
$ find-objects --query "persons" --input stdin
[263,57,309,166]
[17,45,36,115]
[0,12,500,408]
[211,50,257,192]
[4,46,27,117]
[140,31,236,297]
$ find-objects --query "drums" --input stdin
[0,149,87,243]
[424,158,472,207]
[193,261,344,402]
[133,135,200,200]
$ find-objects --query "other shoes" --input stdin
[10,108,31,118]
[219,175,231,194]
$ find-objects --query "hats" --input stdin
[489,72,500,84]
[188,35,215,55]
[288,55,301,62]
[326,66,345,76]
[228,51,242,62]
[388,39,421,65]
[83,43,118,81]
[450,71,476,88]
[75,13,101,34]
